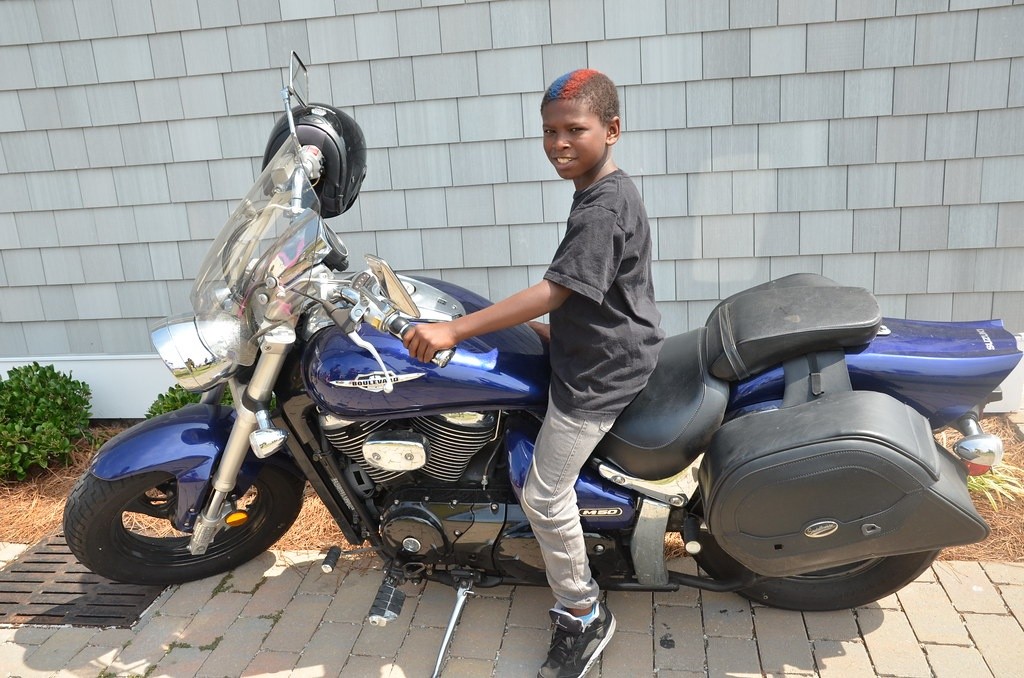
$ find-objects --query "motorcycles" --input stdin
[60,47,1024,678]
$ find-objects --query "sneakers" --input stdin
[537,599,617,678]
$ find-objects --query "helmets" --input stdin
[260,103,366,220]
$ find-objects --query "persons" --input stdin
[402,70,664,678]
